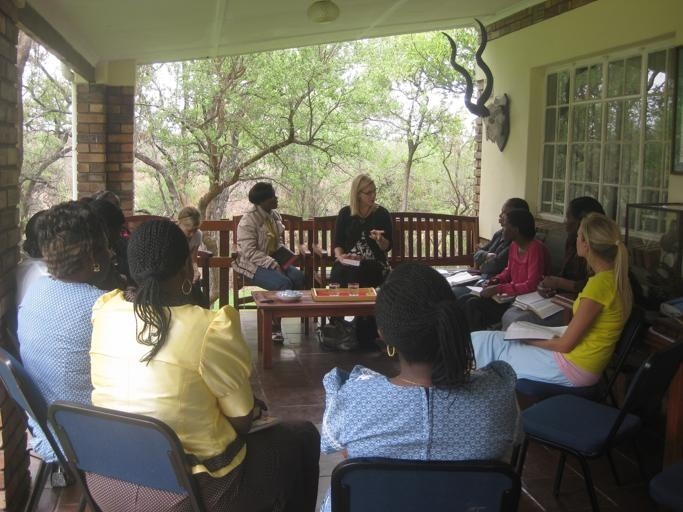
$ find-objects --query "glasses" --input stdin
[360,188,377,196]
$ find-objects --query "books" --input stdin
[343,255,361,267]
[445,271,481,287]
[270,245,298,269]
[466,285,514,304]
[503,320,568,340]
[512,290,566,320]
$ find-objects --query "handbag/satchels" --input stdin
[316,324,354,352]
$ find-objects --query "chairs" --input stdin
[0,348,86,510]
[329,454,520,512]
[44,403,202,512]
[515,310,648,411]
[524,328,683,512]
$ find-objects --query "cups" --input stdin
[325,283,340,296]
[347,282,359,296]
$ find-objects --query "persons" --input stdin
[15,211,54,304]
[502,196,606,329]
[460,209,549,325]
[17,201,115,460]
[330,175,395,337]
[473,198,529,275]
[91,218,321,512]
[231,182,313,343]
[468,213,632,386]
[68,190,132,248]
[322,263,516,512]
[177,206,203,305]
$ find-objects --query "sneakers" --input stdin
[271,331,285,341]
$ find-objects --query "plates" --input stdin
[310,287,377,302]
[274,290,303,302]
